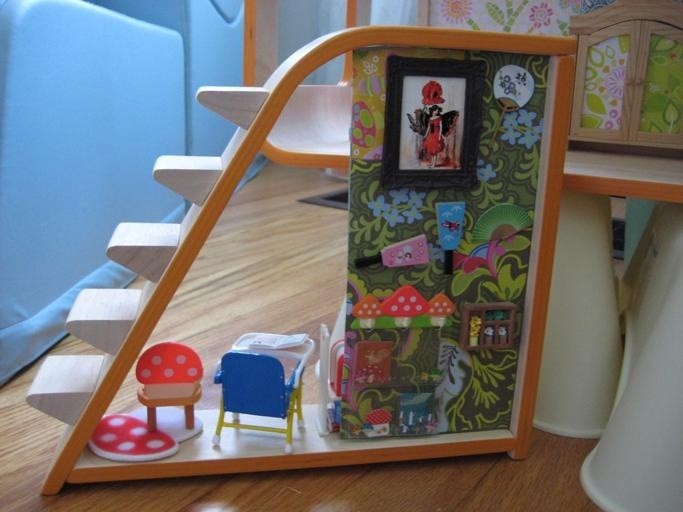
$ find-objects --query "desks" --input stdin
[231,332,314,362]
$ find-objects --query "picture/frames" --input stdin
[377,53,486,191]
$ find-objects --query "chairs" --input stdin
[134,342,204,432]
[210,351,306,455]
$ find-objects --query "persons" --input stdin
[423,107,445,168]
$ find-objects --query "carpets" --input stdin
[292,186,625,262]
